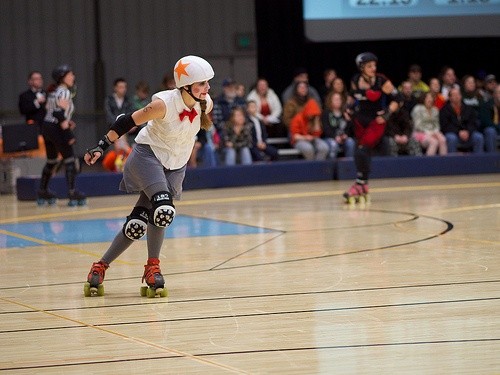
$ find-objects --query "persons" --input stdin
[343,52,405,198]
[83,54,215,285]
[101,62,500,174]
[17,71,49,153]
[36,62,88,201]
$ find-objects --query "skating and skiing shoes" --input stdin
[68,188,87,206]
[140,257,168,297]
[36,190,56,206]
[83,261,108,296]
[343,184,370,204]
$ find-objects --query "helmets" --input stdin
[356,53,377,65]
[52,65,70,82]
[174,55,214,88]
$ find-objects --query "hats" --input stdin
[223,79,236,86]
